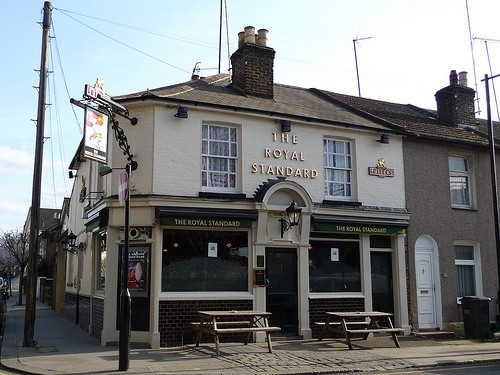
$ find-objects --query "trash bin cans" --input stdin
[459,296,494,341]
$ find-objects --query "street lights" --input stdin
[98,165,130,371]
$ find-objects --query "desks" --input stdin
[318,312,401,350]
[195,311,275,356]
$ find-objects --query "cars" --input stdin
[0,276,6,300]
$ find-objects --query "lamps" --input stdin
[69,171,78,178]
[279,201,302,238]
[67,231,86,252]
[376,134,390,144]
[99,164,130,177]
[62,239,78,255]
[175,107,189,118]
[281,121,292,133]
[81,190,104,200]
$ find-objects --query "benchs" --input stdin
[315,321,406,334]
[190,320,282,334]
